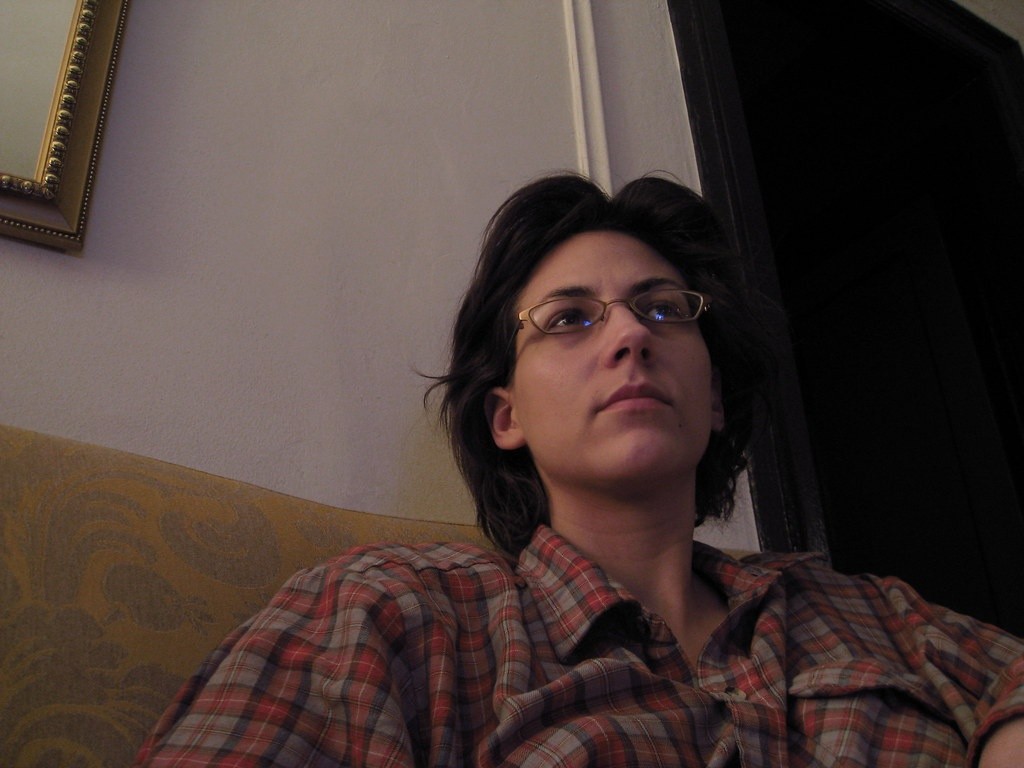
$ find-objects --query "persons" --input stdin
[130,169,1024,768]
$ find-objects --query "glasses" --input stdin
[504,288,713,356]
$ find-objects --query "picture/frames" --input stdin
[0,0,132,255]
